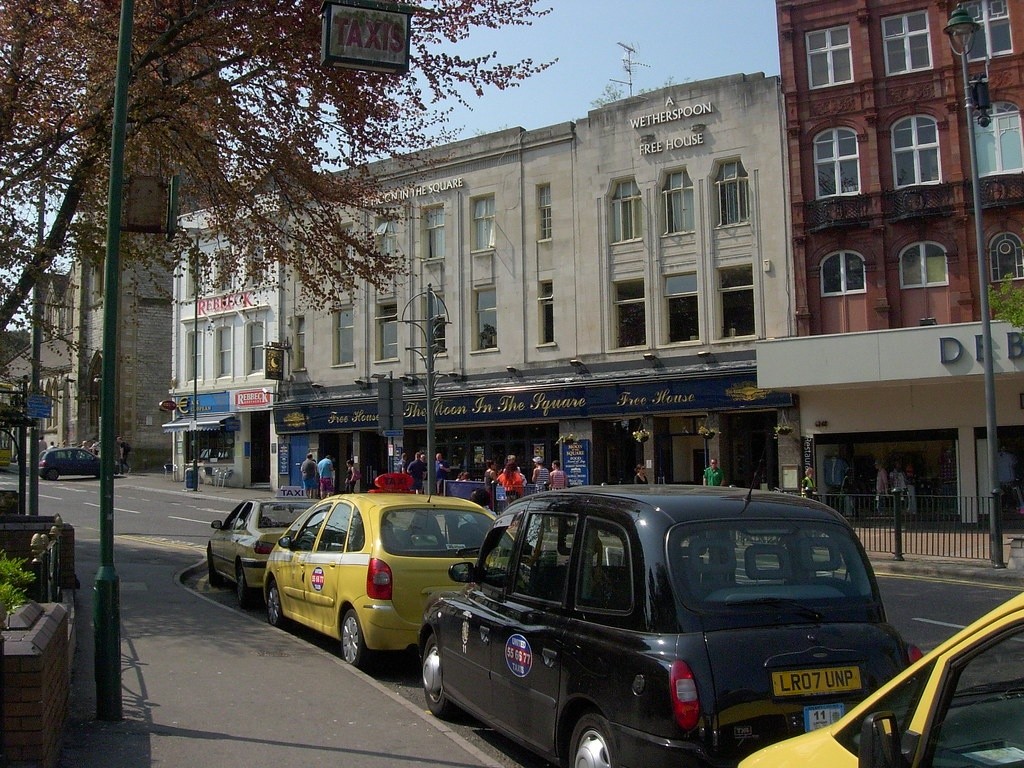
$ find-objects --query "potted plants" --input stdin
[699,412,715,440]
[632,416,649,442]
[554,421,578,445]
[774,408,793,440]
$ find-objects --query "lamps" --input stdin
[697,351,710,364]
[570,360,585,374]
[448,372,460,383]
[311,383,327,394]
[919,317,937,326]
[507,366,519,377]
[354,379,365,390]
[643,353,655,369]
[399,376,414,387]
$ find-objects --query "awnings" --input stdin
[161,416,234,432]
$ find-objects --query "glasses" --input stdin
[711,461,718,465]
[641,468,645,469]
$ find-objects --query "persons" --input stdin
[300,453,321,499]
[116,436,131,474]
[317,454,334,499]
[703,458,725,486]
[531,457,549,492]
[550,460,568,489]
[801,467,820,502]
[822,452,845,513]
[397,453,451,496]
[455,472,470,481]
[49,439,100,455]
[634,464,648,484]
[875,460,917,513]
[39,436,47,453]
[347,460,357,493]
[484,455,527,511]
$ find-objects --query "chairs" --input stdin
[402,513,448,548]
[164,462,232,488]
[454,523,487,546]
[352,517,401,551]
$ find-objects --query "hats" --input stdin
[533,457,543,463]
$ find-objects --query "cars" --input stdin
[736,588,1024,767]
[38,447,100,481]
[263,472,515,669]
[413,484,927,768]
[206,486,323,609]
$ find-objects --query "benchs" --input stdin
[687,536,854,602]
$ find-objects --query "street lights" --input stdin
[942,1,1007,565]
[184,226,203,488]
[399,282,452,495]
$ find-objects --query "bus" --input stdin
[0,427,12,467]
